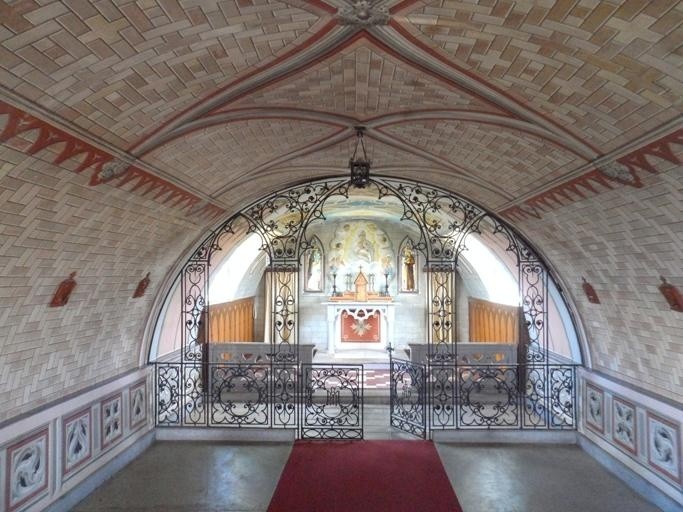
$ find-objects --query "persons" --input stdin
[402,248,415,292]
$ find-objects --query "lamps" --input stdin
[349,126,373,190]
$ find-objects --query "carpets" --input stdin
[266,438,463,511]
[219,367,504,391]
